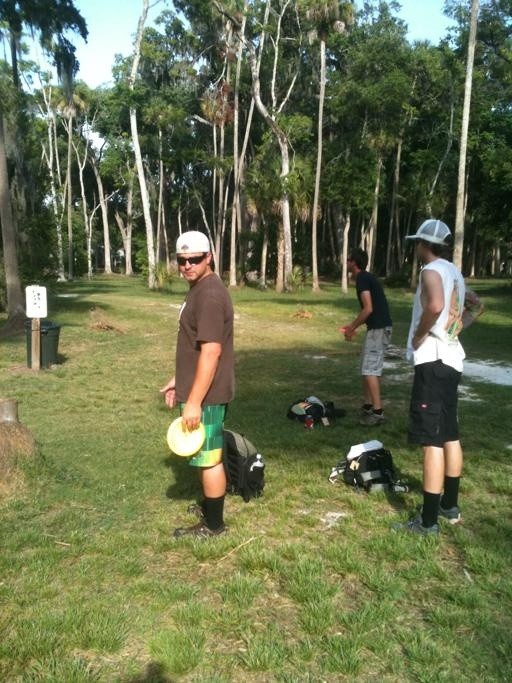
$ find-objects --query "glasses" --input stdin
[175,253,207,265]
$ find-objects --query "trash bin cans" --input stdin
[24,320,61,370]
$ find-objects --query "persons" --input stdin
[158,229,236,539]
[391,218,484,537]
[341,246,394,427]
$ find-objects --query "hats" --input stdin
[404,217,453,246]
[175,230,211,255]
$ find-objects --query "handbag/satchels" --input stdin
[342,440,401,492]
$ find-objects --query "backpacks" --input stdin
[286,395,336,429]
[222,425,266,503]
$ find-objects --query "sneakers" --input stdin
[187,500,207,518]
[172,520,227,541]
[357,404,385,427]
[389,513,443,538]
[416,501,461,523]
